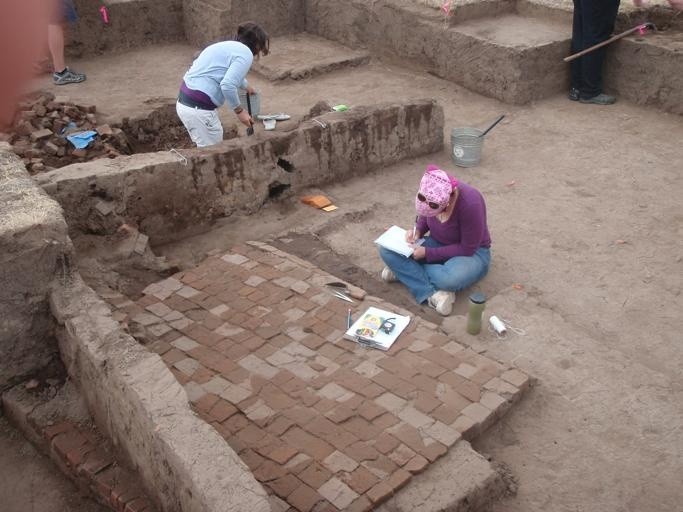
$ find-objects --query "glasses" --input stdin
[418,192,446,209]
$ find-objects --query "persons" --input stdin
[383,164,491,316]
[45,0,86,84]
[176,22,272,148]
[569,0,620,104]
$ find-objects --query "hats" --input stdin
[415,164,458,217]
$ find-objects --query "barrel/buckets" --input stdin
[450,127,483,168]
[237,93,260,120]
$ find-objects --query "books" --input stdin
[375,226,426,257]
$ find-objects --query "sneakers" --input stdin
[381,265,398,281]
[31,57,55,74]
[568,87,579,101]
[579,93,616,104]
[428,290,456,316]
[52,68,86,85]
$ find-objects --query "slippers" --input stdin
[263,117,276,131]
[256,111,291,120]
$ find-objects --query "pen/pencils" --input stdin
[332,290,353,302]
[347,309,351,330]
[413,223,416,244]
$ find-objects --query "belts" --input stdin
[178,98,214,110]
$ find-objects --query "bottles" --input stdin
[468,293,486,336]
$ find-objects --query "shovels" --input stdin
[325,283,364,300]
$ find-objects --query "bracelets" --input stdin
[234,106,245,113]
